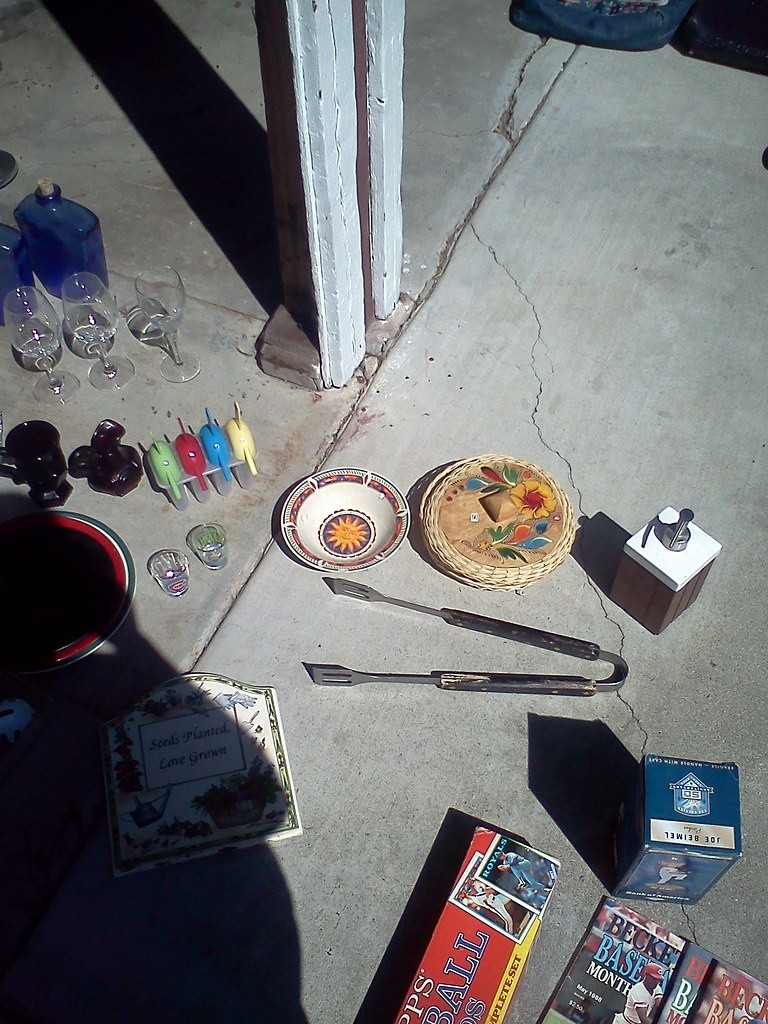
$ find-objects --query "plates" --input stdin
[281,467,411,573]
[0,511,134,676]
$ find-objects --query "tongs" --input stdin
[313,578,628,694]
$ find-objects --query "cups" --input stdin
[192,523,229,571]
[0,420,73,509]
[149,550,188,598]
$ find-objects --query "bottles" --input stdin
[0,223,37,326]
[14,177,109,302]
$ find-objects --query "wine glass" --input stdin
[4,286,81,406]
[62,273,135,392]
[135,266,199,382]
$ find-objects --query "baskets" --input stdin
[420,453,576,596]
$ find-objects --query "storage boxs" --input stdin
[611,754,743,906]
[394,827,562,1024]
